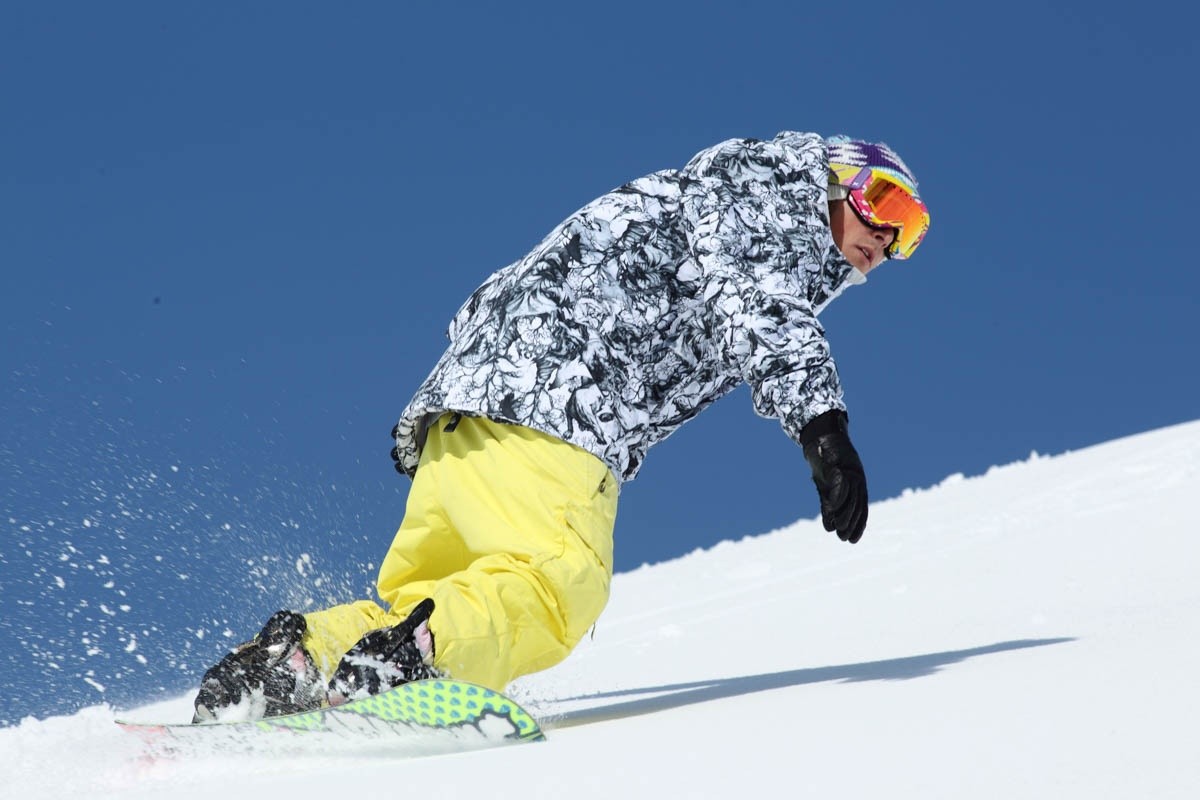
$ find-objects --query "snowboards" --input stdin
[115,677,547,755]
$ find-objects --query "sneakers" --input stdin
[191,611,324,724]
[327,598,440,705]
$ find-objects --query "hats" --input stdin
[824,134,919,202]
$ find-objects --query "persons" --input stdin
[192,129,931,724]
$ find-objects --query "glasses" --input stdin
[846,166,931,261]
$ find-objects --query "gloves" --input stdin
[800,408,869,543]
